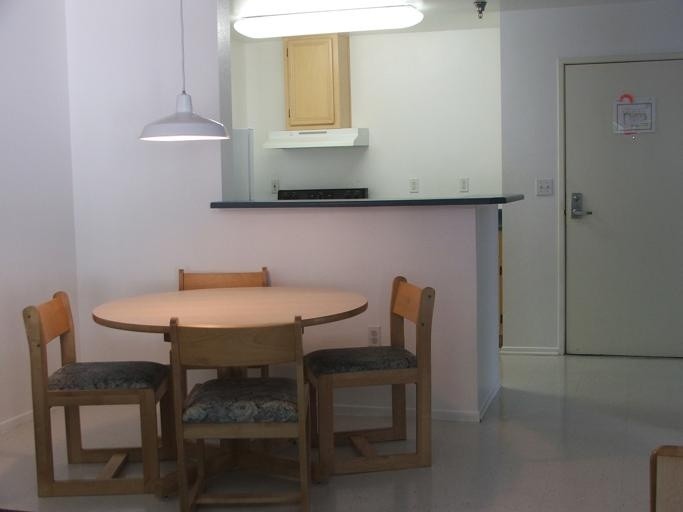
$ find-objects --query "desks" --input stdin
[93,286,369,393]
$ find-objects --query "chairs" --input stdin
[27,291,179,499]
[166,316,314,511]
[177,266,271,379]
[304,272,436,478]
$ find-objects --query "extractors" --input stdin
[264,127,370,149]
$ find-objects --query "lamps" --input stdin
[137,1,229,142]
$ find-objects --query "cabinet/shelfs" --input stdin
[286,37,334,127]
